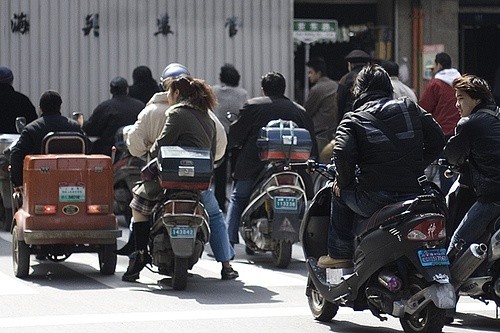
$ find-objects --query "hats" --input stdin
[110,76,129,88]
[40,90,62,105]
[220,64,240,83]
[262,72,285,92]
[1,67,13,84]
[344,50,370,59]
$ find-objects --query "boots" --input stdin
[113,222,135,255]
[130,222,152,276]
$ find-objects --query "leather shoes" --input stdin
[207,254,235,260]
[317,256,353,268]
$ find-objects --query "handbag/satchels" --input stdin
[421,182,451,220]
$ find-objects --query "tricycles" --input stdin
[10,111,122,278]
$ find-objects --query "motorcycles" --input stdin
[111,149,147,228]
[146,145,214,291]
[430,158,500,319]
[224,111,313,269]
[289,158,456,333]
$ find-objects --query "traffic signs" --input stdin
[293,18,339,45]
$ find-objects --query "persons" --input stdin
[300,58,338,154]
[206,72,319,258]
[10,90,92,193]
[441,74,500,323]
[120,62,240,283]
[129,65,159,103]
[212,64,248,213]
[381,62,419,105]
[82,76,146,153]
[0,65,38,133]
[317,63,447,270]
[112,74,217,276]
[338,49,370,121]
[419,52,462,136]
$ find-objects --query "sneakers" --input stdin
[122,271,139,280]
[221,268,238,280]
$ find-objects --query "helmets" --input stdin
[160,63,190,91]
